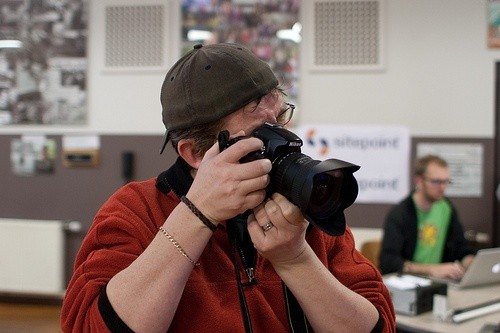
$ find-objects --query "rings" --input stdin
[261,222,273,232]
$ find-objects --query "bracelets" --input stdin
[179,195,217,235]
[157,227,198,269]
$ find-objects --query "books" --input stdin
[380,273,432,291]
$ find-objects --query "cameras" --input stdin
[226,120,361,236]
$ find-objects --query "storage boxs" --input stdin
[383,272,434,317]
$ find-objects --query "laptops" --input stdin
[447,248,500,288]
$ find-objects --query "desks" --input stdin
[395,285,500,333]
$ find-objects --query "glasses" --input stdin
[423,176,451,184]
[275,100,297,127]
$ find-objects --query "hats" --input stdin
[158,42,279,155]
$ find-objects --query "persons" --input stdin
[379,155,482,284]
[61,43,396,333]
